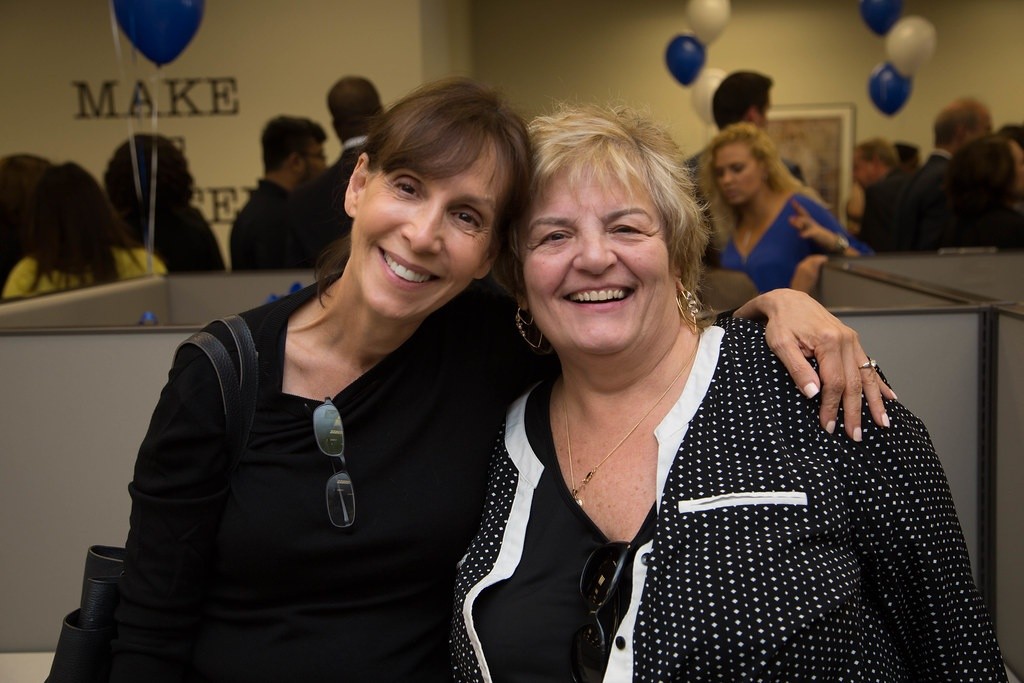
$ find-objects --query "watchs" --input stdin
[834,232,849,254]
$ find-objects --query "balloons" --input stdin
[665,33,705,86]
[885,16,936,78]
[860,0,904,36]
[686,0,730,47]
[869,63,913,118]
[691,69,728,126]
[111,0,204,68]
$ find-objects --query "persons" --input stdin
[282,74,385,269]
[110,65,898,683]
[0,154,169,301]
[229,113,329,274]
[449,90,1010,683]
[681,72,806,187]
[104,132,229,272]
[698,121,872,295]
[846,95,1024,256]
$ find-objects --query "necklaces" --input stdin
[561,334,700,506]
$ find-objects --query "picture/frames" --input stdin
[765,105,856,236]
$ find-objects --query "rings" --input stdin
[858,359,877,370]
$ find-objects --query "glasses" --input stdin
[312,396,356,528]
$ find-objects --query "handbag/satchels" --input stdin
[47,315,257,683]
